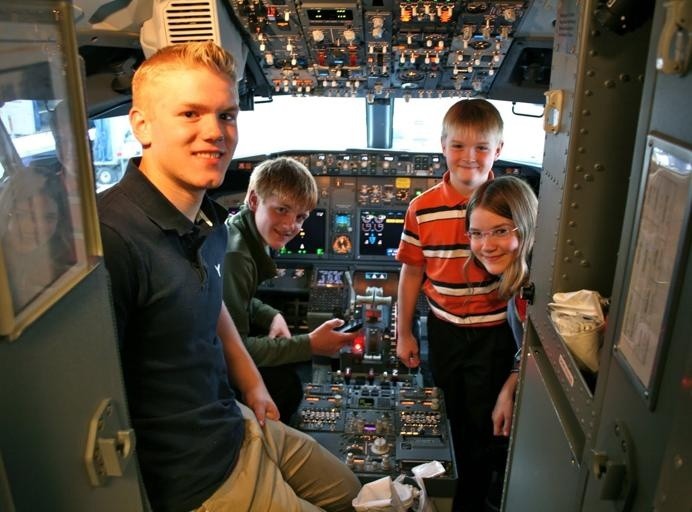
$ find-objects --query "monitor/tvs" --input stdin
[358,210,406,256]
[279,208,325,254]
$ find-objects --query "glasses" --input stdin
[464,226,519,240]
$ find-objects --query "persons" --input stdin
[0,166,74,316]
[462,176,538,436]
[97,40,362,512]
[225,156,363,423]
[396,101,519,512]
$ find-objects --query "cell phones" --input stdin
[339,319,364,332]
